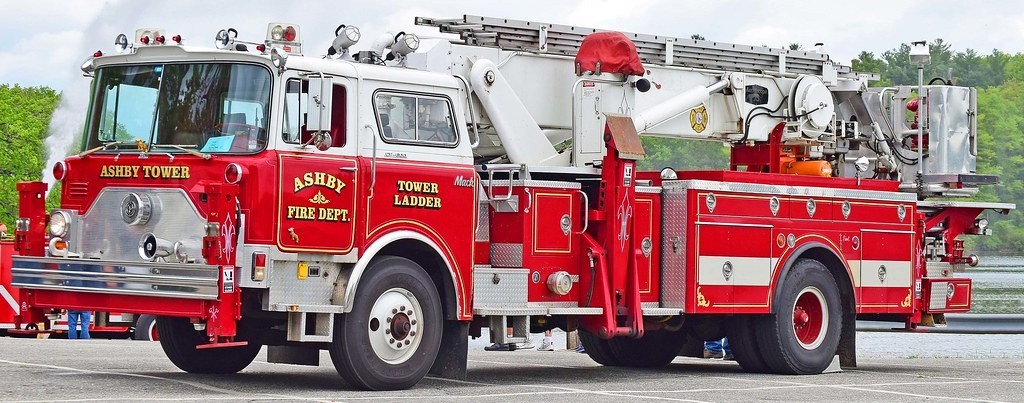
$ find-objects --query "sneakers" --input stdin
[517,337,536,349]
[537,337,555,351]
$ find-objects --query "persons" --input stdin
[67,309,92,339]
[484,327,588,354]
[704,337,736,361]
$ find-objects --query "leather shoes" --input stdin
[484,342,517,351]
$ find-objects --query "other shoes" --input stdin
[576,343,585,353]
[703,349,735,361]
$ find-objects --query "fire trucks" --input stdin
[10,15,1016,390]
[0,233,160,341]
